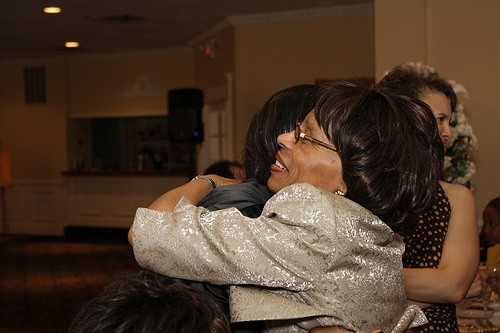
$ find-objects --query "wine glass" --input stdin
[478,263,498,327]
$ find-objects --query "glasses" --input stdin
[294,119,341,154]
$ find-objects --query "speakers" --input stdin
[169,88,204,142]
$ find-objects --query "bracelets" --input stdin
[190,175,217,190]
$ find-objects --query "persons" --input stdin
[69,265,232,333]
[128,61,500,333]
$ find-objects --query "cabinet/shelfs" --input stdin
[0,176,189,237]
